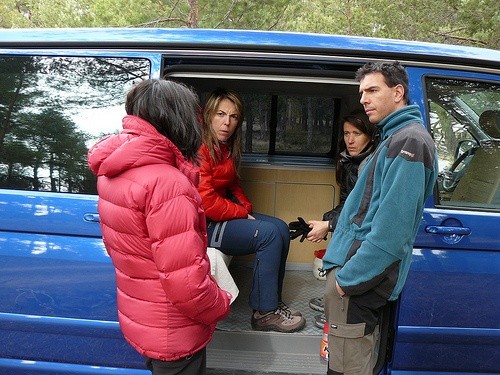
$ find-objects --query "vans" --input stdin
[0,27,500,375]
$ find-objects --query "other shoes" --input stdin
[315,313,325,328]
[309,295,324,312]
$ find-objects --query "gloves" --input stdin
[288,216,328,243]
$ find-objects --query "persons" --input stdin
[324,60,439,374]
[87,78,231,374]
[193,88,306,333]
[307,107,377,327]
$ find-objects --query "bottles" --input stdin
[319,322,329,365]
[313,249,327,280]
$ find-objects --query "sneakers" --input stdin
[252,309,304,333]
[279,300,302,316]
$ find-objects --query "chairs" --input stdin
[451,110,500,205]
